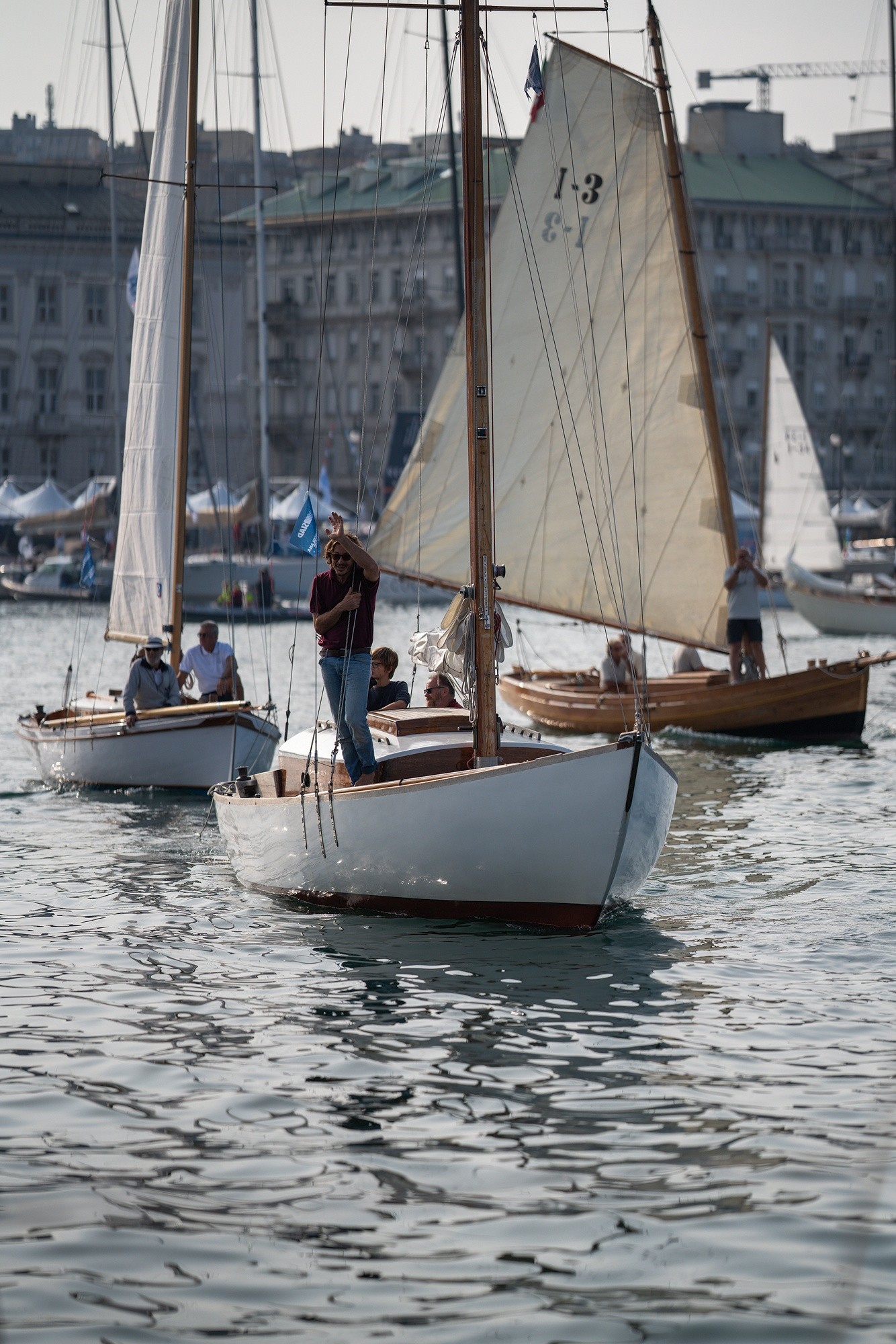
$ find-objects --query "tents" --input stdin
[0,479,349,546]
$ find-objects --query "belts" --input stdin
[319,648,371,657]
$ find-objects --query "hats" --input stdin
[142,636,169,650]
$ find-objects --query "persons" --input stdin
[724,548,769,684]
[600,634,644,692]
[424,674,464,708]
[54,529,64,555]
[103,527,112,559]
[367,647,410,712]
[309,512,380,786]
[672,644,710,673]
[80,527,88,542]
[177,620,244,714]
[217,567,275,608]
[123,636,181,728]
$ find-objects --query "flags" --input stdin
[79,541,96,588]
[288,494,322,559]
[317,469,332,505]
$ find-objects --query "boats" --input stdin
[781,544,896,636]
[181,604,313,620]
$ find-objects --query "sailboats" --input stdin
[0,0,451,597]
[17,0,282,786]
[761,329,843,606]
[356,0,896,744]
[213,0,680,932]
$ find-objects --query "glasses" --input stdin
[146,647,161,652]
[424,686,445,694]
[331,552,352,562]
[611,646,623,652]
[371,662,383,668]
[197,632,214,638]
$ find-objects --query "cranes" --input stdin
[697,59,890,112]
